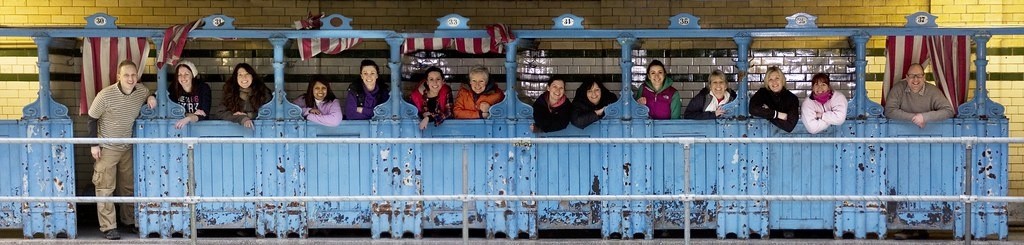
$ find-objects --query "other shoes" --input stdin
[104,229,121,239]
[122,223,138,233]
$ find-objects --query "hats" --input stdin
[173,61,199,79]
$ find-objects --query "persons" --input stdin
[344,59,390,120]
[801,73,848,134]
[571,76,618,129]
[88,60,157,240]
[407,66,454,130]
[749,66,799,132]
[214,63,273,130]
[884,63,954,128]
[684,69,737,120]
[166,59,212,130]
[453,64,504,118]
[529,74,571,133]
[636,59,681,119]
[291,73,342,127]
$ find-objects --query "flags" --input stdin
[157,18,237,70]
[293,15,362,61]
[881,36,970,116]
[79,36,150,117]
[400,38,453,54]
[455,23,516,54]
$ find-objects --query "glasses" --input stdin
[908,73,925,79]
